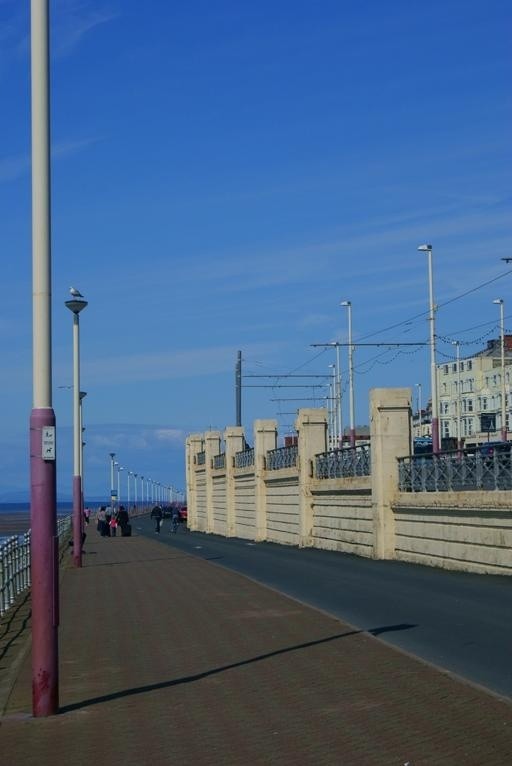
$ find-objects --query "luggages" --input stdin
[122,523,131,536]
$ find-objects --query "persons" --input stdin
[159,504,164,527]
[170,506,180,533]
[151,504,161,536]
[67,531,86,554]
[83,503,130,538]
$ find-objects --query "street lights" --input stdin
[451,340,462,451]
[65,286,89,569]
[491,298,506,441]
[415,382,421,436]
[320,299,355,452]
[107,452,187,521]
[419,241,440,455]
